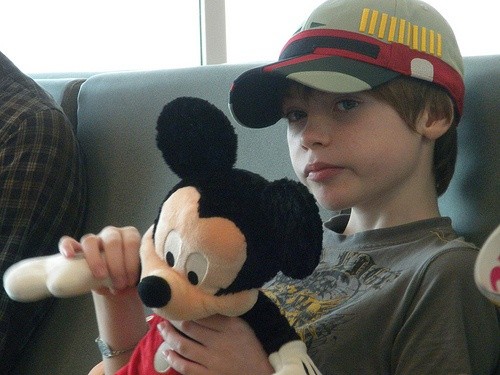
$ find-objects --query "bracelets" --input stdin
[94,336,137,360]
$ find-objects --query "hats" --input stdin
[228,0,464,129]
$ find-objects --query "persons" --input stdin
[59,0,500,375]
[0,54,86,375]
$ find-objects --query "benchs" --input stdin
[13,54,500,375]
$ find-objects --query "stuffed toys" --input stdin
[3,98,323,375]
